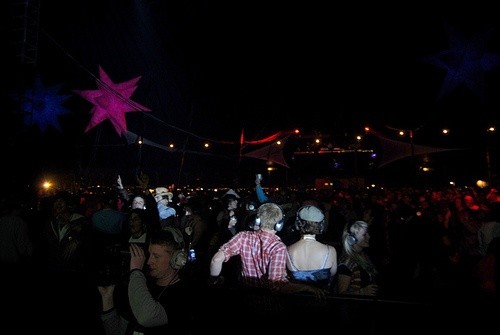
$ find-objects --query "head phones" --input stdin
[254,202,283,232]
[346,220,357,245]
[165,227,188,270]
[294,206,326,233]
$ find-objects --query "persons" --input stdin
[98,231,212,335]
[33,173,500,300]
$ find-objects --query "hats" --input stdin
[154,187,174,203]
[300,205,325,222]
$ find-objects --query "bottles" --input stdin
[190,250,196,261]
